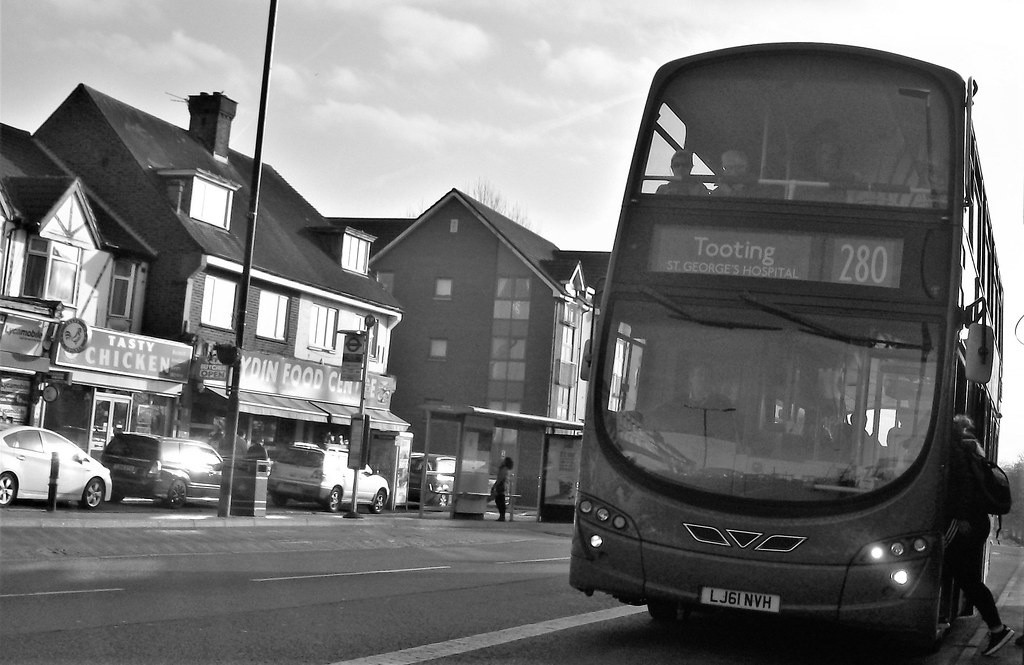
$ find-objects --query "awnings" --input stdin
[206,387,411,431]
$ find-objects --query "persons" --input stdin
[944,415,1014,655]
[656,149,763,198]
[209,429,268,460]
[491,457,514,522]
[658,361,734,441]
[819,409,911,450]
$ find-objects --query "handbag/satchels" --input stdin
[496,482,505,493]
[947,458,1011,515]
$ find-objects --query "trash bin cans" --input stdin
[230,457,274,518]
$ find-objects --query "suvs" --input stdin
[405,451,510,511]
[101,431,223,510]
[266,438,391,514]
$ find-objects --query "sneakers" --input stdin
[938,616,950,629]
[981,624,1016,656]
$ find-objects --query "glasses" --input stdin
[671,162,691,168]
[722,163,744,171]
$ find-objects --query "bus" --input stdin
[567,42,1010,652]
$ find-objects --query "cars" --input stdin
[0,421,113,511]
